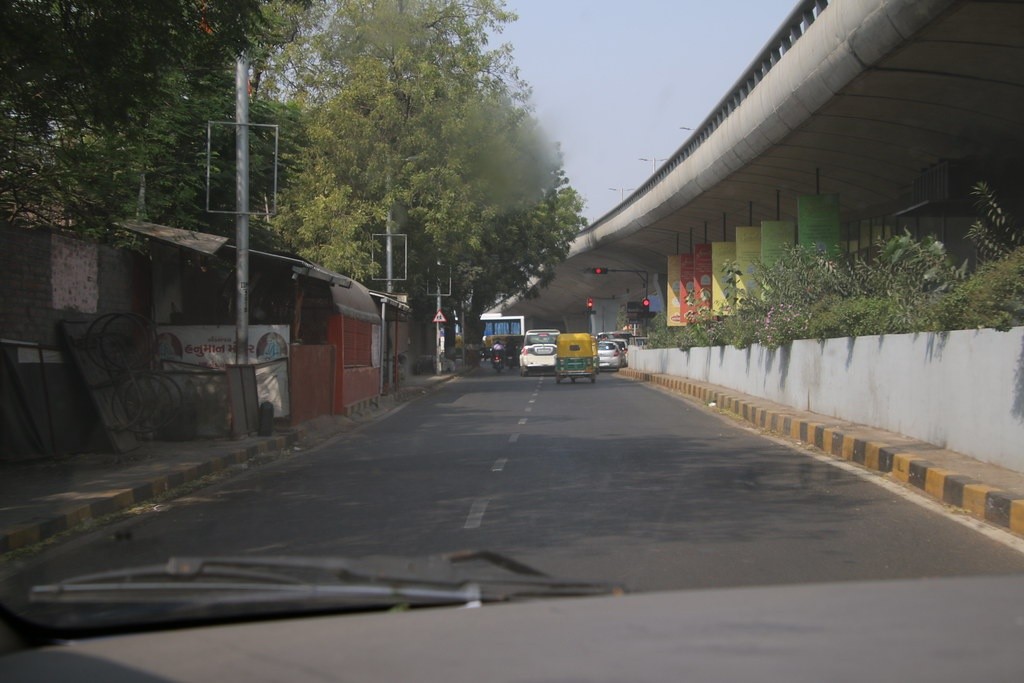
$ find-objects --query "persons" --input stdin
[494,341,505,350]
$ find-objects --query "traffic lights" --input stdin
[642,298,650,307]
[590,268,608,274]
[586,297,593,309]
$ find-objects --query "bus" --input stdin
[438,310,526,353]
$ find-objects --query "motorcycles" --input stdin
[555,330,599,385]
[488,346,505,373]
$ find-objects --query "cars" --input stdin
[628,336,647,345]
[595,331,632,341]
[519,329,561,376]
[609,338,628,366]
[597,340,626,371]
